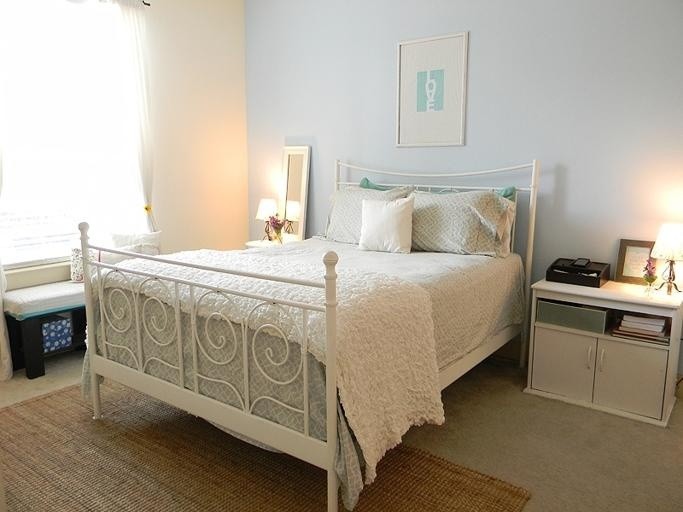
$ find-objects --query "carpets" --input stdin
[0,381,531,512]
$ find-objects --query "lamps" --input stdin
[255,198,277,240]
[648,222,683,295]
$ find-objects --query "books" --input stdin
[612,313,670,347]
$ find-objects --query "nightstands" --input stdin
[244,238,293,248]
[523,278,683,428]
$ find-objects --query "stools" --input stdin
[4,278,85,380]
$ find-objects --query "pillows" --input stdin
[326,185,414,245]
[407,190,508,258]
[70,243,142,282]
[356,196,414,253]
[110,230,162,255]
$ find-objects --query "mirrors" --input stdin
[279,145,312,241]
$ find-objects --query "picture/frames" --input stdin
[613,238,657,286]
[394,30,467,147]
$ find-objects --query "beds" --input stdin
[79,157,540,512]
